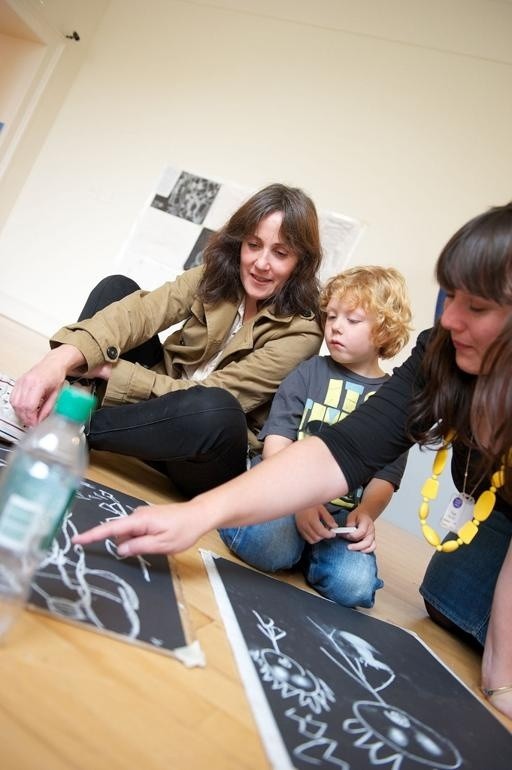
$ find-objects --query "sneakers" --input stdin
[0,372,44,448]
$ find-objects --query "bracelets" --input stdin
[480,684,512,699]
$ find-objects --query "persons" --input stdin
[210,260,419,613]
[8,182,330,499]
[65,195,511,729]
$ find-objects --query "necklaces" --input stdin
[418,423,512,555]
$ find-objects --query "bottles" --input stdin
[0,385,98,645]
